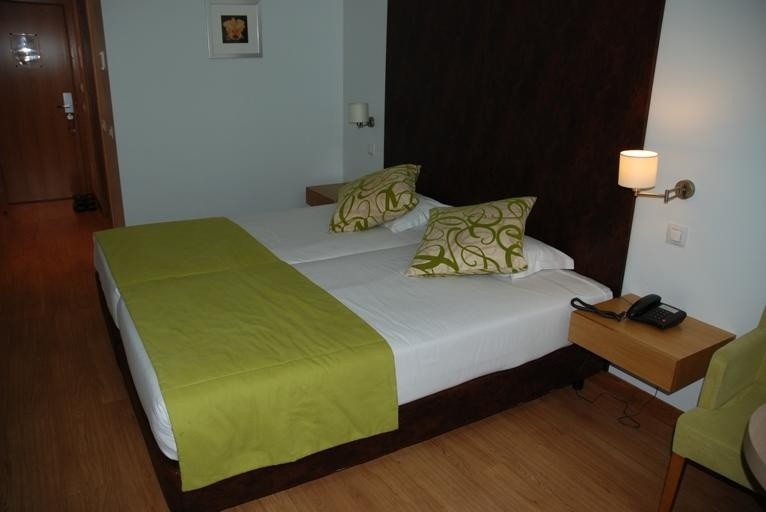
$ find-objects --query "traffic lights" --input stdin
[330,162,573,282]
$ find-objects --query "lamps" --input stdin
[662,308,765,512]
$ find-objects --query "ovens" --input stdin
[92,193,621,510]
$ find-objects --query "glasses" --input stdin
[348,102,376,129]
[614,147,695,203]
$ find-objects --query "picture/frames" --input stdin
[570,295,737,395]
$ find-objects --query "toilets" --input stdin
[627,294,687,329]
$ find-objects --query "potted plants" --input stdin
[206,2,263,58]
